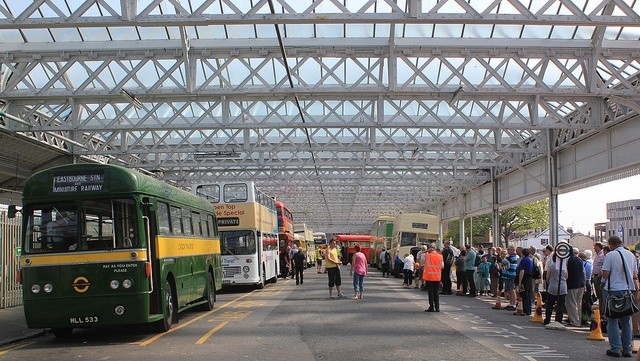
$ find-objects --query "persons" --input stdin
[413,262,420,288]
[630,250,640,338]
[402,253,414,288]
[602,245,610,256]
[323,244,329,274]
[291,247,306,286]
[477,241,593,330]
[350,244,367,300]
[340,240,347,265]
[593,242,605,300]
[288,244,299,280]
[379,248,393,278]
[464,244,476,297]
[326,238,345,299]
[43,211,77,249]
[599,234,637,357]
[419,243,444,312]
[315,245,322,275]
[336,240,342,264]
[440,240,454,295]
[453,250,466,296]
[280,246,291,278]
[416,245,426,288]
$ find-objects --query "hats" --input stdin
[584,249,592,258]
[573,248,579,252]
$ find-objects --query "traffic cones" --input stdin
[513,297,526,316]
[620,316,639,354]
[492,290,504,309]
[529,296,545,322]
[587,307,606,341]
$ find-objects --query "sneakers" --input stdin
[507,305,516,310]
[504,305,509,308]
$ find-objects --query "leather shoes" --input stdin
[606,349,620,356]
[436,309,439,312]
[623,352,632,356]
[425,306,434,311]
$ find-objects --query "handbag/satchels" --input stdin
[605,292,640,317]
[385,251,391,261]
[475,254,481,266]
[532,266,541,279]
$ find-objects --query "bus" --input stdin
[367,215,394,268]
[293,224,316,269]
[335,233,372,266]
[293,232,306,269]
[390,211,440,277]
[376,221,393,271]
[311,231,327,259]
[275,201,294,276]
[8,163,225,335]
[192,180,280,289]
